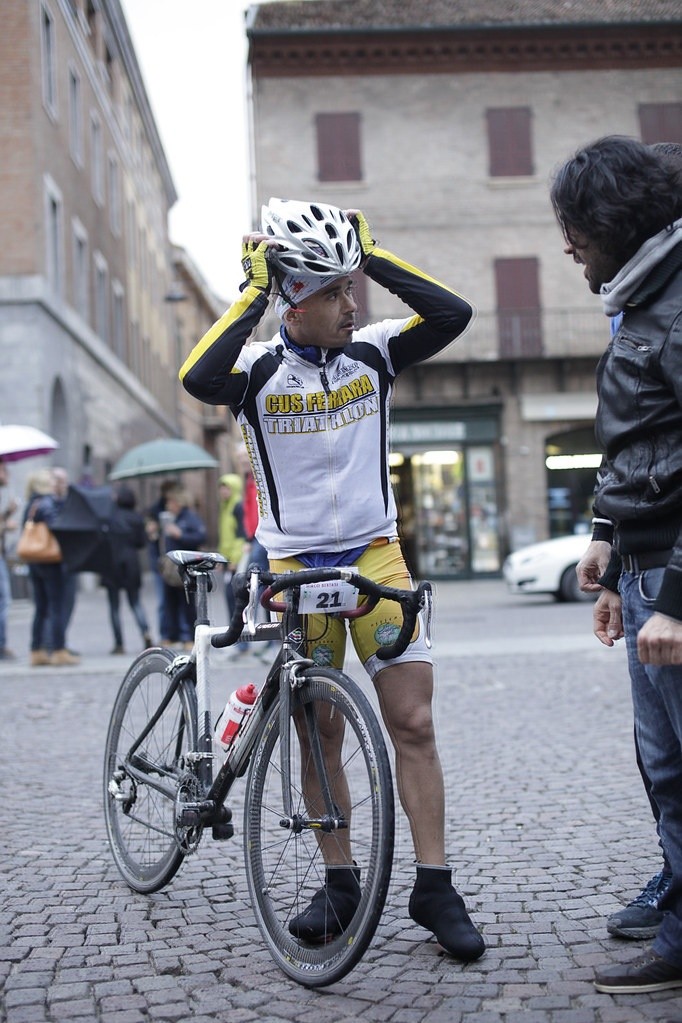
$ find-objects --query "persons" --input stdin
[177,195,486,960]
[551,133,682,994]
[224,472,283,663]
[143,479,207,650]
[1,470,21,658]
[96,485,153,655]
[22,467,82,667]
[216,474,245,627]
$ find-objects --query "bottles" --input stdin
[213,683,258,750]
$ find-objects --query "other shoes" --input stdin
[114,647,125,653]
[52,649,80,665]
[144,634,153,650]
[32,649,51,664]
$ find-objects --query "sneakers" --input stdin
[289,876,361,942]
[607,866,682,940]
[592,949,680,993]
[410,881,485,963]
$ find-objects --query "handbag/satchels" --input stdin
[18,505,63,565]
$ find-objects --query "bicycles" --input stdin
[102,549,434,988]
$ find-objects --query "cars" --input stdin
[502,534,603,604]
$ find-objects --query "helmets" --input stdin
[262,196,361,277]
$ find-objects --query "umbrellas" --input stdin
[49,483,131,576]
[0,425,61,463]
[106,438,223,481]
